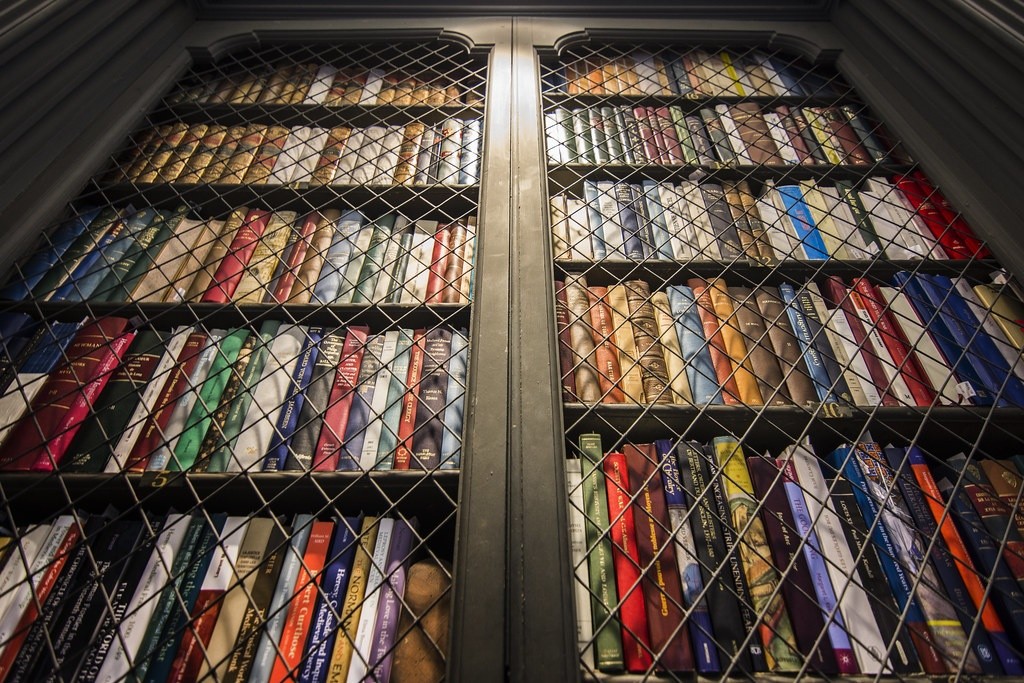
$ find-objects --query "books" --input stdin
[3,15,1024,683]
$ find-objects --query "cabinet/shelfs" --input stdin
[0,0,1024,683]
